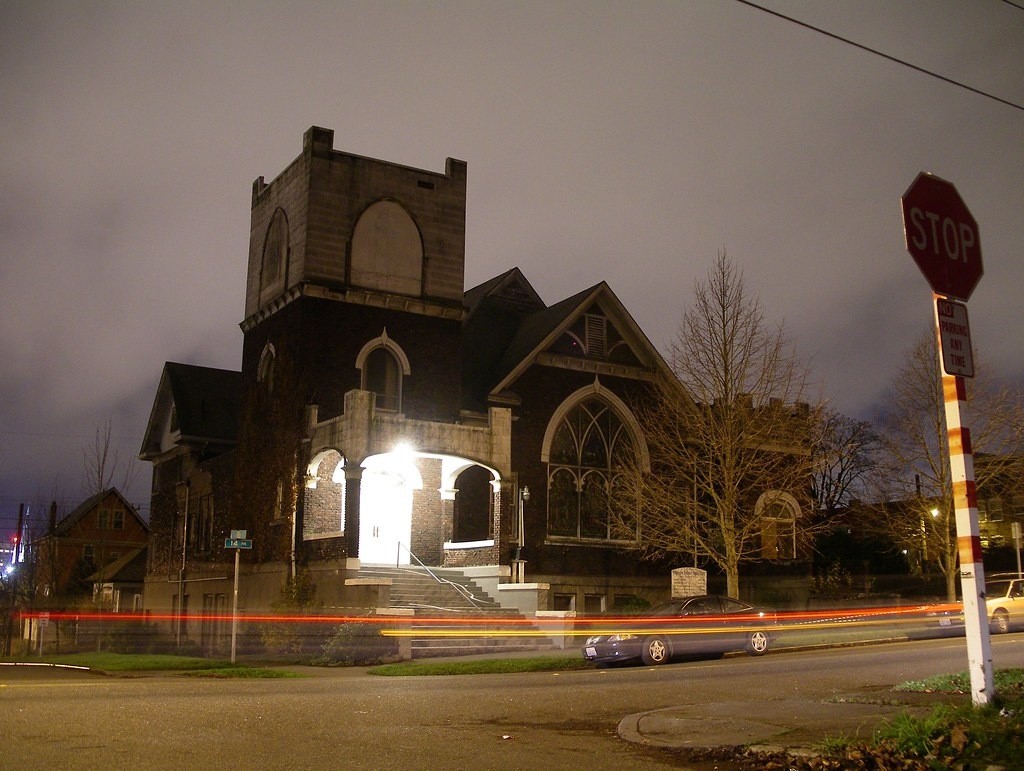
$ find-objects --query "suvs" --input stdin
[926,570,1023,635]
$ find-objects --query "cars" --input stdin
[581,595,782,666]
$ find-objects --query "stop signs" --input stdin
[901,172,984,303]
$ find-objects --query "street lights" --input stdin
[914,474,939,577]
